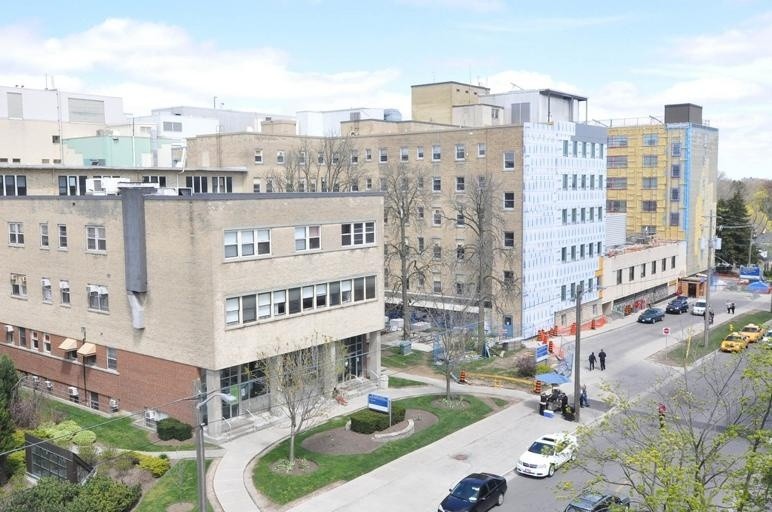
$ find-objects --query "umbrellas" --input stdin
[534,373,572,393]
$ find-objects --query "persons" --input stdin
[579,384,590,409]
[331,387,349,407]
[731,300,737,314]
[599,349,606,371]
[657,400,666,428]
[589,352,597,371]
[560,393,568,416]
[725,299,731,313]
[540,391,551,415]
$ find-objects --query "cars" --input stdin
[438,472,507,512]
[564,489,632,512]
[720,324,772,354]
[638,296,711,324]
[517,432,578,479]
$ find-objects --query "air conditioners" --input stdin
[110,399,118,407]
[11,273,97,294]
[45,381,52,389]
[68,387,78,396]
[4,324,13,332]
[145,411,153,419]
[32,376,40,383]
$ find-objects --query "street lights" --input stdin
[186,377,239,512]
[574,285,607,423]
